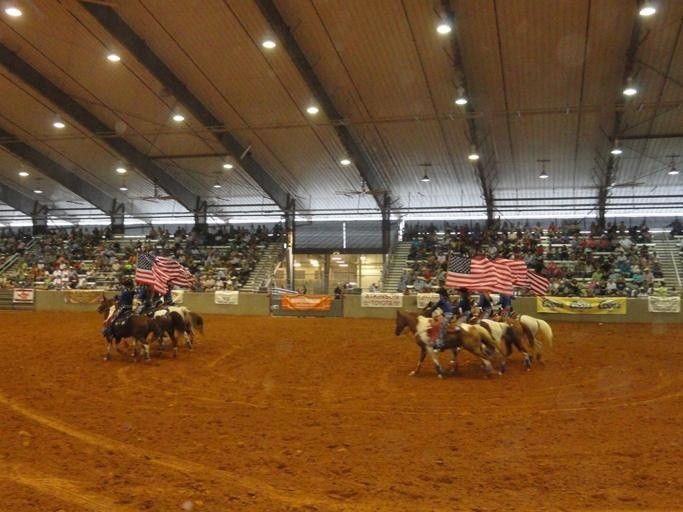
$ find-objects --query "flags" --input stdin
[526,269,549,300]
[444,254,499,288]
[134,251,196,296]
[466,257,513,296]
[496,255,528,290]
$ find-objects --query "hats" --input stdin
[456,287,469,293]
[435,287,449,297]
[122,279,134,288]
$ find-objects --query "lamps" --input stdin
[211,176,222,190]
[434,9,481,162]
[609,0,659,158]
[417,163,432,182]
[536,161,550,178]
[666,153,680,175]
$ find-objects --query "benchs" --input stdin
[0,230,283,297]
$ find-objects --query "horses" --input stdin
[395,310,498,379]
[421,301,514,376]
[132,298,195,349]
[452,299,534,372]
[491,302,553,366]
[95,294,167,362]
[169,304,203,345]
[113,296,186,359]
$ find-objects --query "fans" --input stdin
[22,199,49,217]
[331,177,392,196]
[577,175,645,193]
[127,177,181,201]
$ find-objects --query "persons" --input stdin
[493,293,516,312]
[664,217,682,237]
[268,223,283,241]
[102,279,135,340]
[134,284,152,315]
[426,287,457,348]
[334,285,341,299]
[152,289,161,308]
[396,220,664,297]
[163,285,173,305]
[374,285,379,292]
[454,287,473,323]
[0,222,267,292]
[369,282,375,292]
[473,291,493,319]
